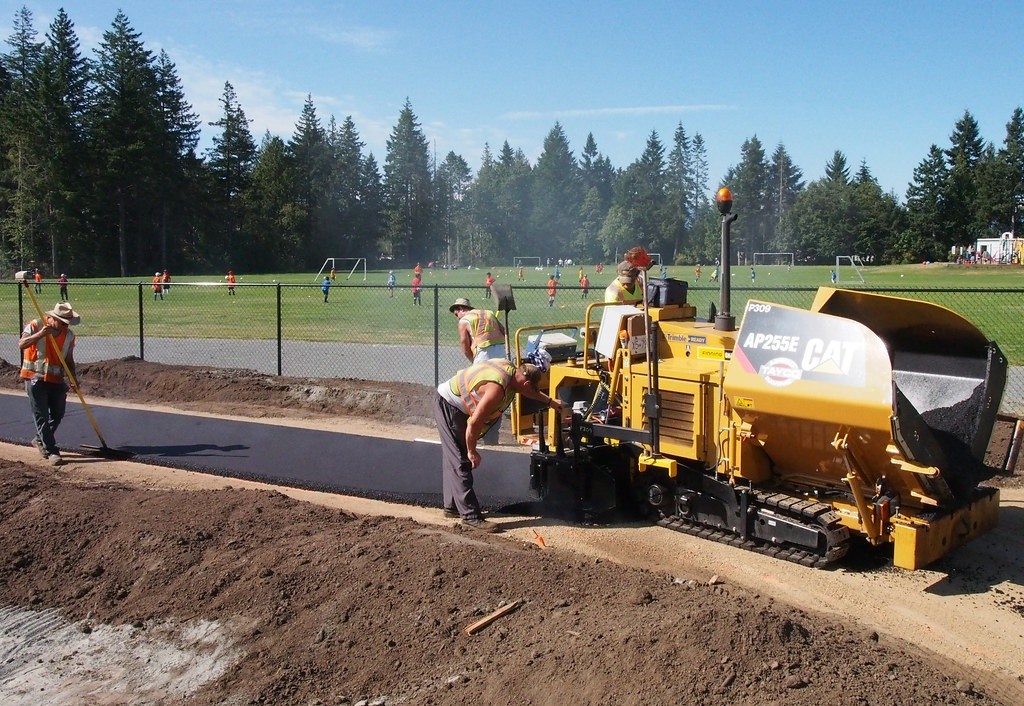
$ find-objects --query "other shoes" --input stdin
[463,518,498,532]
[444,507,460,518]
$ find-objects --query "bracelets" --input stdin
[545,398,552,405]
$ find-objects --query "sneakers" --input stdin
[49,454,62,467]
[31,436,47,457]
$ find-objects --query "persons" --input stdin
[546,258,569,308]
[485,271,495,299]
[578,267,589,299]
[751,267,755,284]
[595,263,604,275]
[20,302,82,464]
[153,269,171,301]
[34,269,42,294]
[694,264,701,282]
[225,271,236,295]
[58,274,68,301]
[387,271,396,298]
[321,267,335,302]
[518,267,525,281]
[411,262,423,307]
[830,270,837,283]
[605,262,644,304]
[450,298,506,364]
[432,357,565,532]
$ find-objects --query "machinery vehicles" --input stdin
[511,189,1008,572]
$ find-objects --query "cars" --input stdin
[379,253,394,260]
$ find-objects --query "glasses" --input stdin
[57,318,69,327]
[455,309,459,318]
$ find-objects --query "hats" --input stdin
[228,270,232,274]
[449,298,475,312]
[61,274,66,277]
[521,363,542,393]
[155,272,161,276]
[45,301,80,325]
[617,261,638,283]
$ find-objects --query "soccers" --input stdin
[224,275,229,279]
[600,272,603,275]
